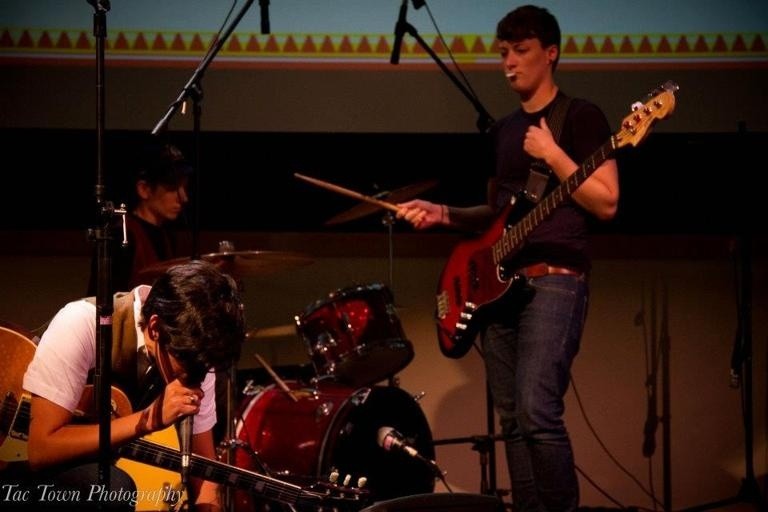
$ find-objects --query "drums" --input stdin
[214,377,435,512]
[294,282,414,386]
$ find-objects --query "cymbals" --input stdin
[321,180,437,226]
[243,325,298,344]
[135,250,314,275]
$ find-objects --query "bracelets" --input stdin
[439,204,445,225]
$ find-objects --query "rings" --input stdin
[187,394,196,403]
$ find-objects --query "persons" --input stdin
[87,141,196,298]
[394,6,624,510]
[21,261,249,511]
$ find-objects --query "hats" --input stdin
[137,142,196,183]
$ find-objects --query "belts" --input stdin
[514,260,573,277]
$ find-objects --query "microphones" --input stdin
[375,426,441,476]
[178,414,193,486]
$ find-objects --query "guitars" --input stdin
[433,81,679,359]
[0,323,369,512]
